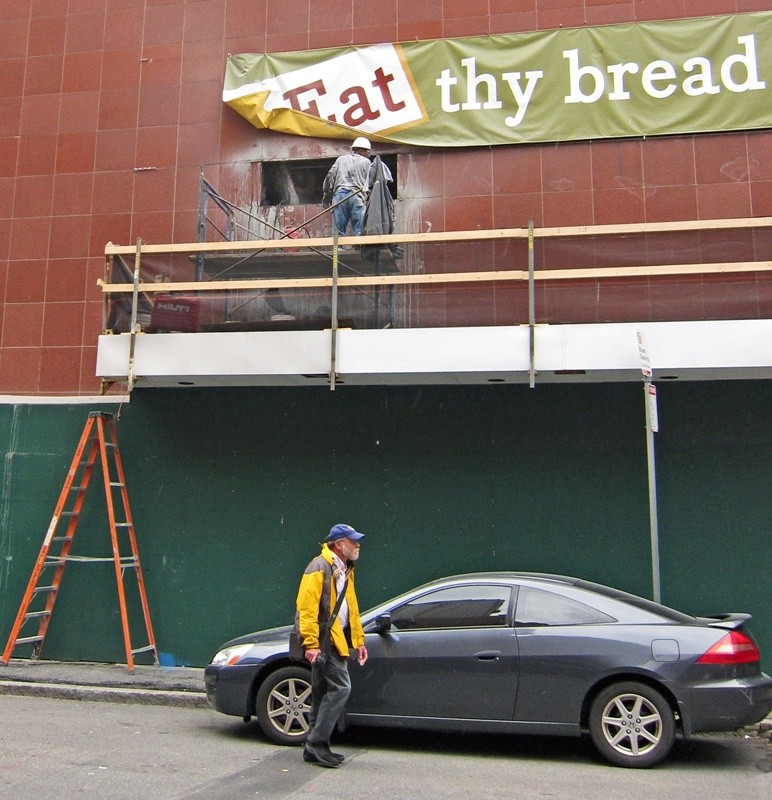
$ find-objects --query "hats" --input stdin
[327,523,366,541]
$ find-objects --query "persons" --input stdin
[322,137,374,250]
[294,525,369,768]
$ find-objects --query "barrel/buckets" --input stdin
[270,315,296,321]
[127,309,151,333]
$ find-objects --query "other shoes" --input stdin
[338,246,345,252]
[305,741,338,766]
[303,750,344,763]
[352,246,360,251]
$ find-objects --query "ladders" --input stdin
[1,412,159,676]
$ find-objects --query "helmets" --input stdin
[350,137,372,151]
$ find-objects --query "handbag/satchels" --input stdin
[289,621,330,664]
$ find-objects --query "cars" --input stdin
[203,569,771,768]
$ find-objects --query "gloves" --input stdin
[322,195,332,209]
